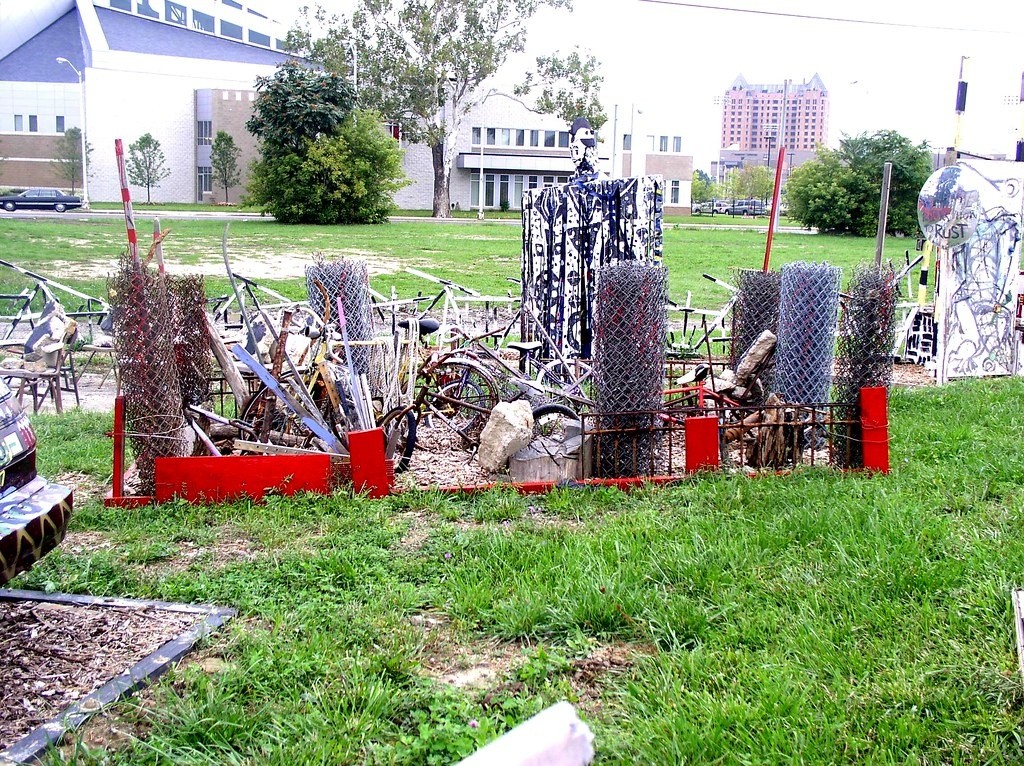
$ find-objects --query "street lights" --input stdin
[722,165,727,183]
[788,153,796,175]
[629,103,642,179]
[340,39,358,124]
[55,56,91,210]
[477,88,498,219]
[763,136,776,206]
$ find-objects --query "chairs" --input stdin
[0,316,121,415]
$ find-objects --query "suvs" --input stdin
[726,200,766,216]
[692,202,726,213]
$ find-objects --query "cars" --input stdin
[0,190,82,212]
[0,381,75,593]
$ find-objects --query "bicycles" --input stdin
[235,304,765,473]
